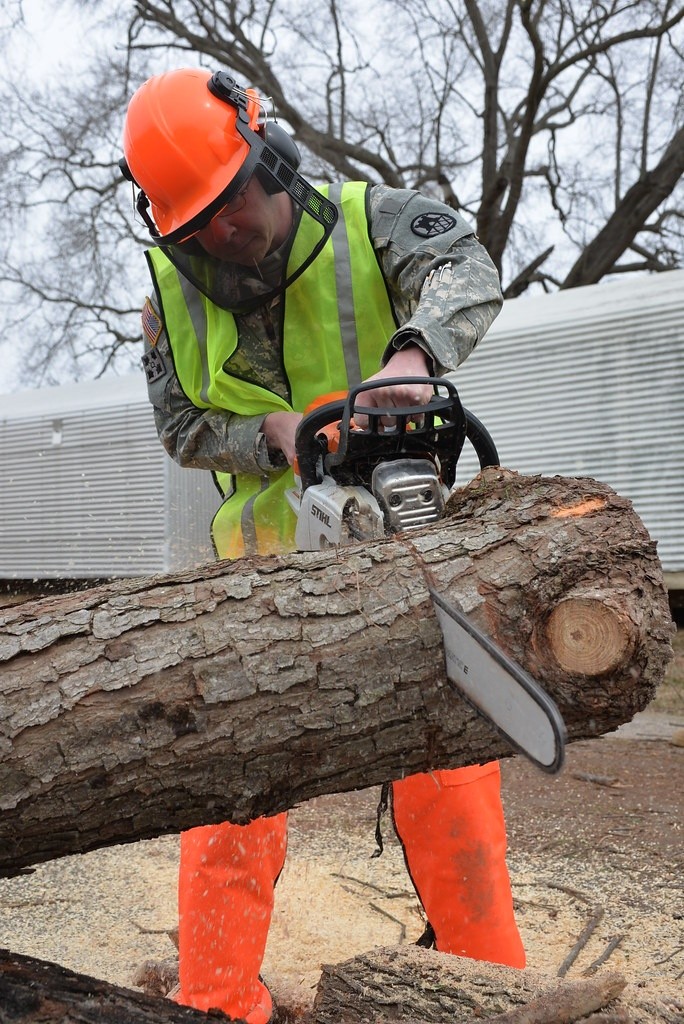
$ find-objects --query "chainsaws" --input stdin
[279,382,570,773]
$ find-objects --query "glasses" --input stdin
[218,172,255,217]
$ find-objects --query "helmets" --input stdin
[123,68,262,244]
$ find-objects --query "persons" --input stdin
[118,68,525,1024]
